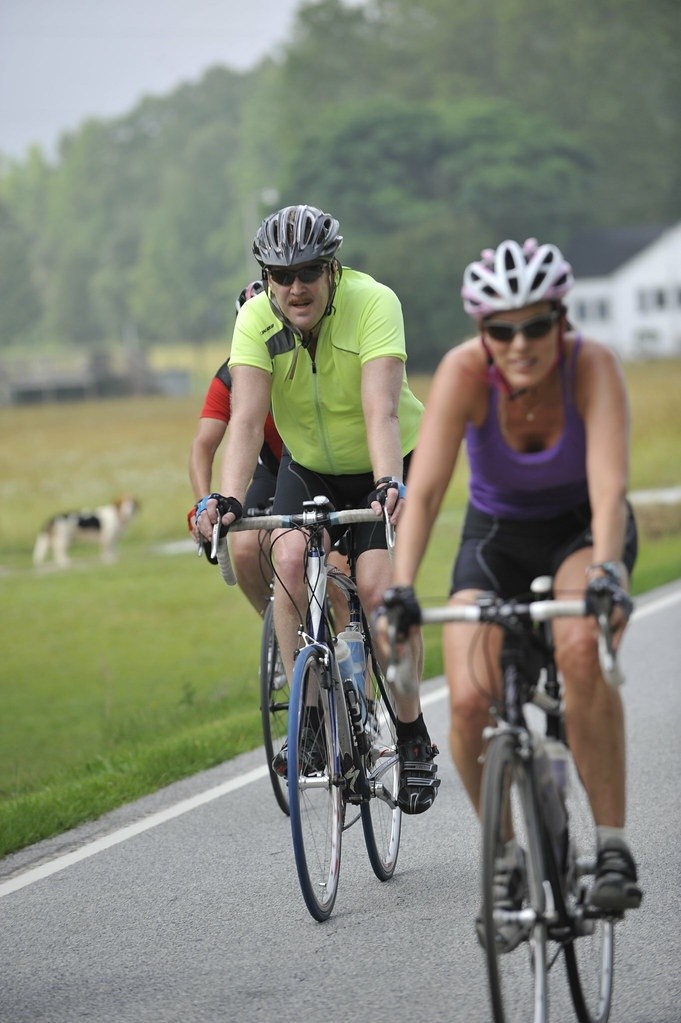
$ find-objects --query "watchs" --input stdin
[584,561,622,581]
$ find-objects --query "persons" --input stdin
[196,203,442,815]
[370,236,643,956]
[188,280,371,703]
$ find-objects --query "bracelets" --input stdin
[374,476,397,489]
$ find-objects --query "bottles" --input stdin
[332,625,365,698]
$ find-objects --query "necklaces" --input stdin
[515,396,544,421]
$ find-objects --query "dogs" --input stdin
[33,499,142,564]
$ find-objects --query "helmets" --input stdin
[462,237,575,320]
[234,280,265,315]
[250,204,342,269]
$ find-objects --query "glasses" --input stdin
[266,262,330,287]
[479,312,561,342]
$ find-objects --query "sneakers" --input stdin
[591,842,642,910]
[395,728,441,814]
[475,843,526,951]
[272,722,328,777]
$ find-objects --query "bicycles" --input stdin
[374,583,614,1023]
[191,491,408,922]
